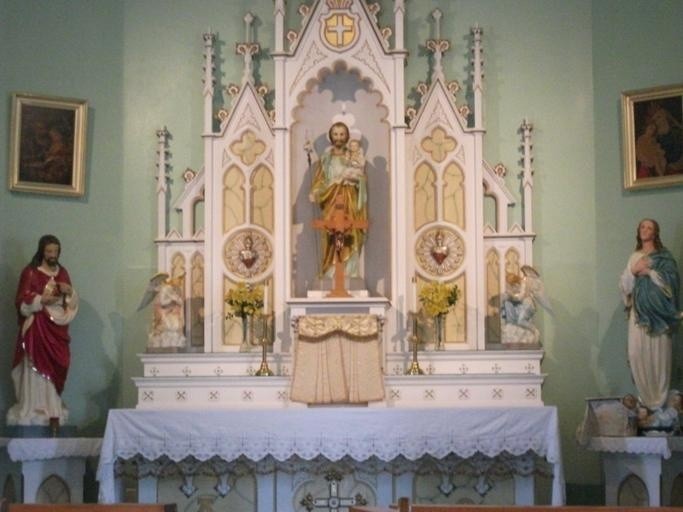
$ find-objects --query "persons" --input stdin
[307,122,370,281]
[430,231,449,263]
[16,121,68,184]
[642,100,683,166]
[238,237,258,268]
[334,139,364,187]
[9,234,78,438]
[36,126,65,168]
[151,276,183,330]
[618,217,683,413]
[500,273,539,343]
[620,391,682,429]
[634,121,666,180]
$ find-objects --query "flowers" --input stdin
[223,280,263,342]
[418,281,462,347]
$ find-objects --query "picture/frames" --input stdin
[8,89,90,196]
[619,82,683,191]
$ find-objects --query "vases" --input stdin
[239,316,252,352]
[432,313,446,352]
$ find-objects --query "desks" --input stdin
[94,403,565,512]
